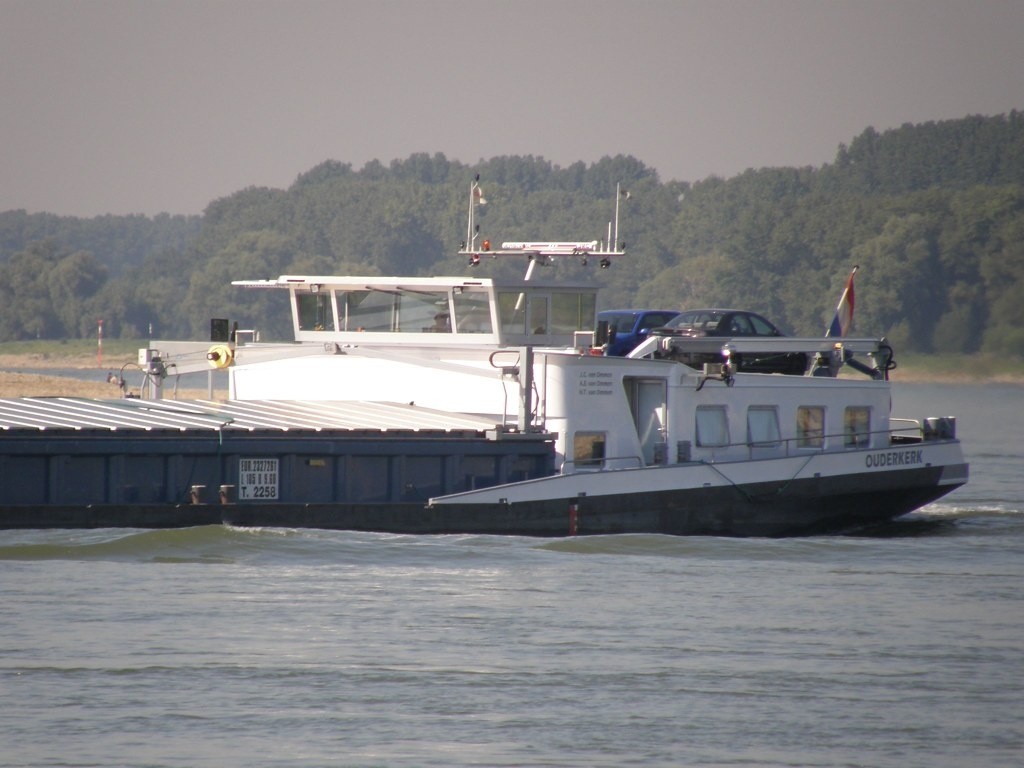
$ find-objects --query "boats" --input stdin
[206,174,970,538]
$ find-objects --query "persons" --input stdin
[430,313,451,333]
[106,372,119,385]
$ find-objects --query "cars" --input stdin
[651,308,809,377]
[596,308,682,358]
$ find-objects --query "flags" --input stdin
[814,274,855,377]
